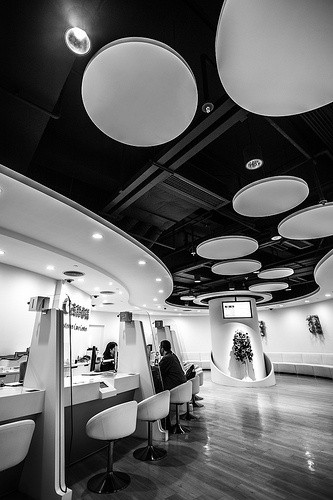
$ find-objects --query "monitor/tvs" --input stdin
[222,300,253,319]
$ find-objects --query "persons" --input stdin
[99,341,119,371]
[150,339,187,433]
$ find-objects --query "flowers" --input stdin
[257,321,265,337]
[232,329,254,365]
[306,314,323,336]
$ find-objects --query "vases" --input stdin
[241,358,254,383]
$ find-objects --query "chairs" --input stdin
[84,401,137,495]
[133,375,192,462]
[0,419,35,471]
[182,361,204,409]
